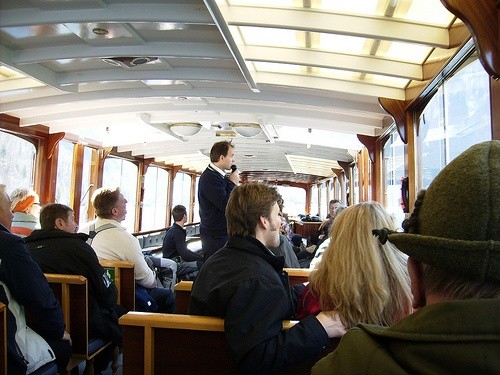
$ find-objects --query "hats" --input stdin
[372,140,500,275]
[9,187,40,212]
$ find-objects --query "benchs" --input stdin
[294,222,322,239]
[0,261,325,375]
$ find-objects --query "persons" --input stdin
[21,203,127,375]
[297,232,324,260]
[7,186,42,238]
[294,203,418,354]
[75,186,176,315]
[162,204,204,282]
[291,234,304,257]
[311,199,341,232]
[311,140,500,375]
[183,185,351,375]
[198,142,240,267]
[0,184,72,375]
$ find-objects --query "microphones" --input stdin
[231,165,237,172]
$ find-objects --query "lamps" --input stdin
[232,124,262,137]
[170,123,202,137]
